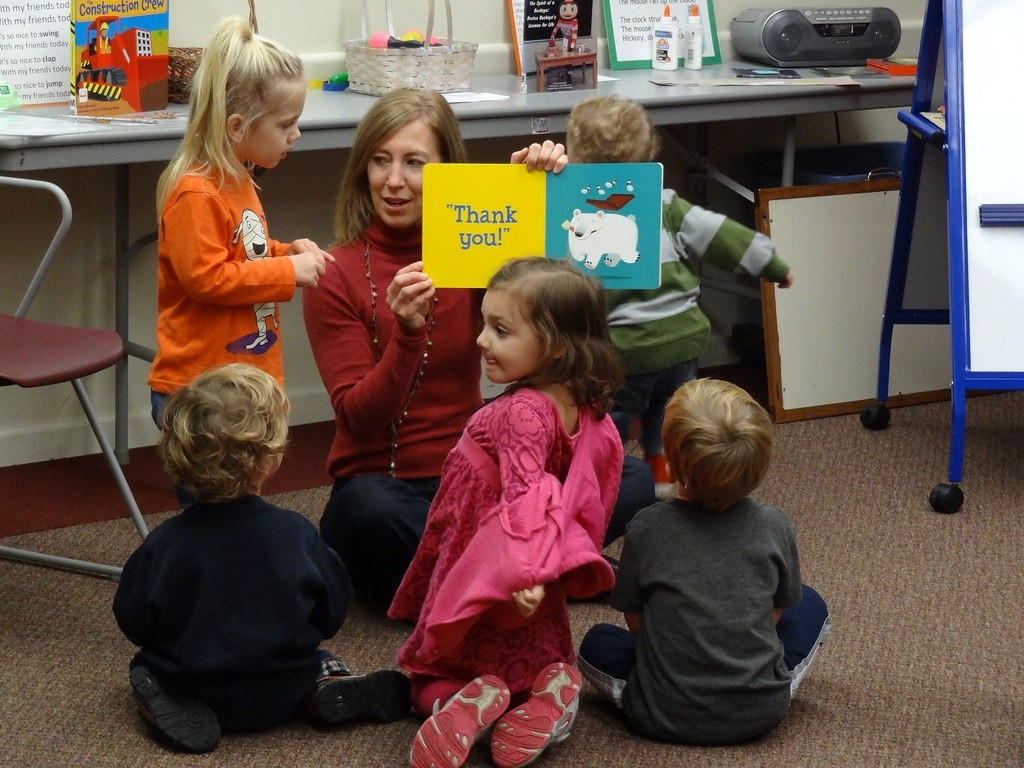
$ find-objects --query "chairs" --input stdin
[0,174,147,579]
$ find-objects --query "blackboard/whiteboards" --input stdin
[754,178,1018,424]
[885,0,1023,393]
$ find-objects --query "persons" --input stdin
[566,99,794,492]
[577,378,830,744]
[388,258,624,768]
[551,0,578,83]
[147,20,336,512]
[116,363,412,754]
[301,87,653,604]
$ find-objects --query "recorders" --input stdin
[730,7,901,68]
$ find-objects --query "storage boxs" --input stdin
[745,138,908,183]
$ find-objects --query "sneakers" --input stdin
[410,673,511,768]
[491,663,583,768]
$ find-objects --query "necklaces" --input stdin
[365,244,438,477]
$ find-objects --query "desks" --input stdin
[1,54,920,463]
[534,46,597,92]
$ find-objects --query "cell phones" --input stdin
[734,68,802,80]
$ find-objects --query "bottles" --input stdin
[652,4,679,71]
[683,3,702,70]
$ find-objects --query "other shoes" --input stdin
[312,670,413,726]
[644,452,672,498]
[131,661,222,753]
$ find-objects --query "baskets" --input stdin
[344,0,479,98]
